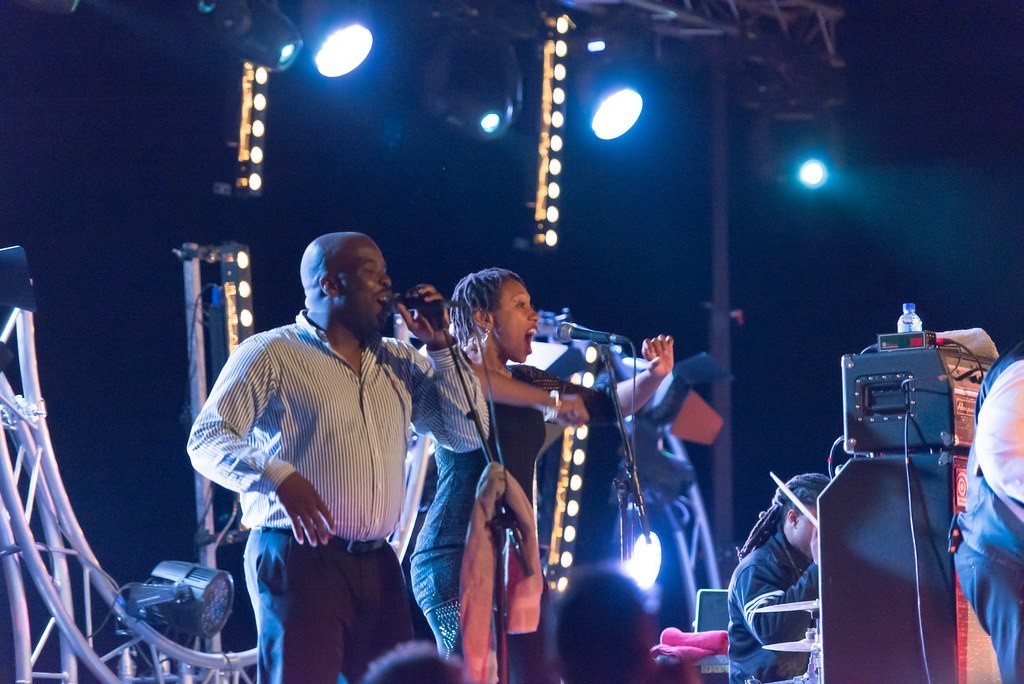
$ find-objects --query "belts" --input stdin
[253,525,387,553]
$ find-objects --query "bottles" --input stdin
[898,303,922,334]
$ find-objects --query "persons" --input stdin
[409,269,672,684]
[952,341,1024,684]
[547,569,659,684]
[725,474,831,684]
[186,231,489,684]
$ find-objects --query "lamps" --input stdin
[422,36,523,139]
[127,560,235,639]
[189,1,304,72]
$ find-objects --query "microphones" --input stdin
[556,322,630,344]
[389,292,468,314]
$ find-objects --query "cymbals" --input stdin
[761,638,820,652]
[748,599,818,613]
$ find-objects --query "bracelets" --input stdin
[544,392,561,424]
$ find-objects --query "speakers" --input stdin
[815,452,1003,684]
